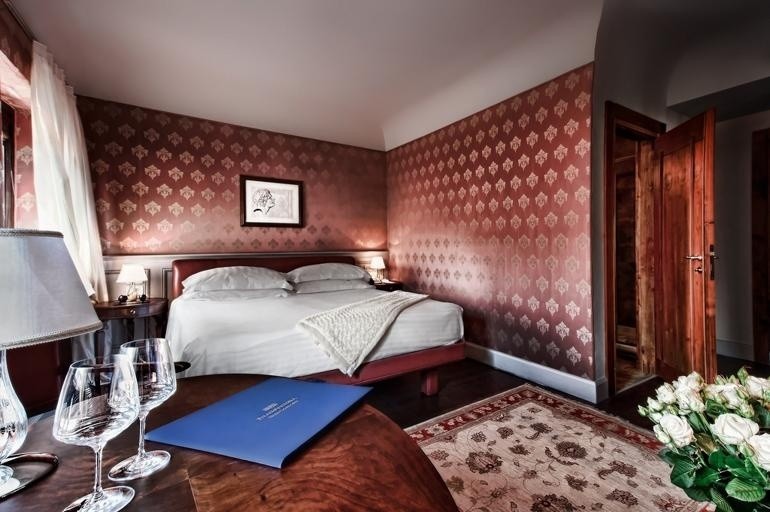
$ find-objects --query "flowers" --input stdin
[635,364,770,512]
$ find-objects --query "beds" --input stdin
[172,257,466,394]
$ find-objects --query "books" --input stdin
[143,375,376,470]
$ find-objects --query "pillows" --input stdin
[182,262,377,301]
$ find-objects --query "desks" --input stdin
[374,280,403,291]
[95,299,169,396]
[1,373,463,512]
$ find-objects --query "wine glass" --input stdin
[108,337,180,485]
[0,347,28,498]
[52,354,138,512]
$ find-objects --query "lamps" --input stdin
[0,228,103,461]
[116,263,148,301]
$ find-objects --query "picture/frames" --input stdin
[239,175,304,227]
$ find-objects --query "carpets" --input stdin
[402,385,719,512]
